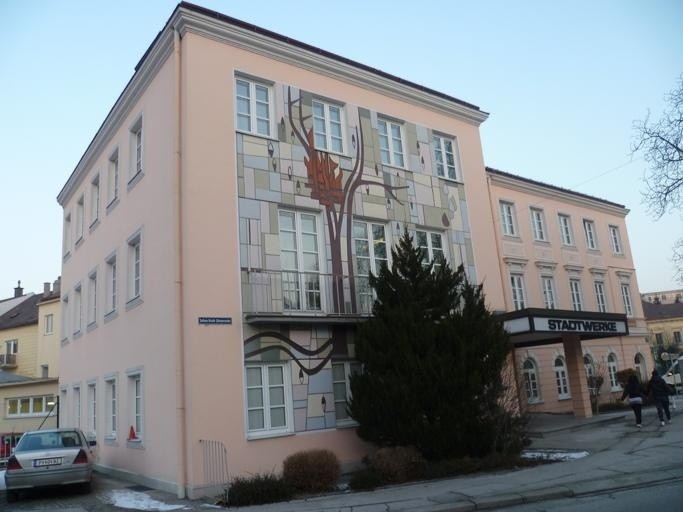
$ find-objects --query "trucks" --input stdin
[662,357,683,393]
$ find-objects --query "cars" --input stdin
[4,428,102,497]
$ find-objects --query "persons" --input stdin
[645,370,674,426]
[621,375,646,428]
